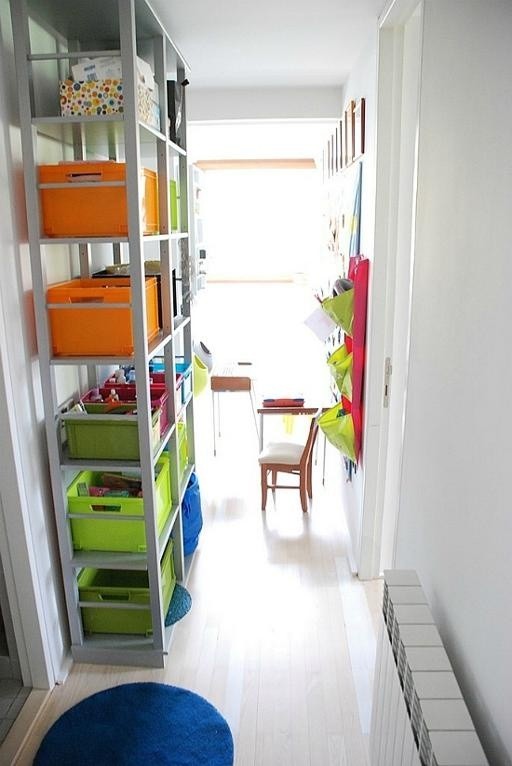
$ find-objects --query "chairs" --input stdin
[210,375,259,456]
[257,406,323,512]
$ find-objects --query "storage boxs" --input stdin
[35,160,159,237]
[80,387,172,437]
[66,457,173,553]
[59,80,161,131]
[62,403,163,459]
[160,418,190,481]
[120,357,192,404]
[168,180,179,233]
[182,471,203,556]
[103,372,183,422]
[32,276,160,357]
[75,266,178,331]
[75,538,177,637]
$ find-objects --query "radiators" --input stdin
[369,566,489,765]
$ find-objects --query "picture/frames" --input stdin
[322,97,365,181]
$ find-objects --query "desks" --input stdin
[257,405,333,466]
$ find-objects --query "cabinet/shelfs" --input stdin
[7,1,195,671]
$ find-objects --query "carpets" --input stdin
[31,681,234,766]
